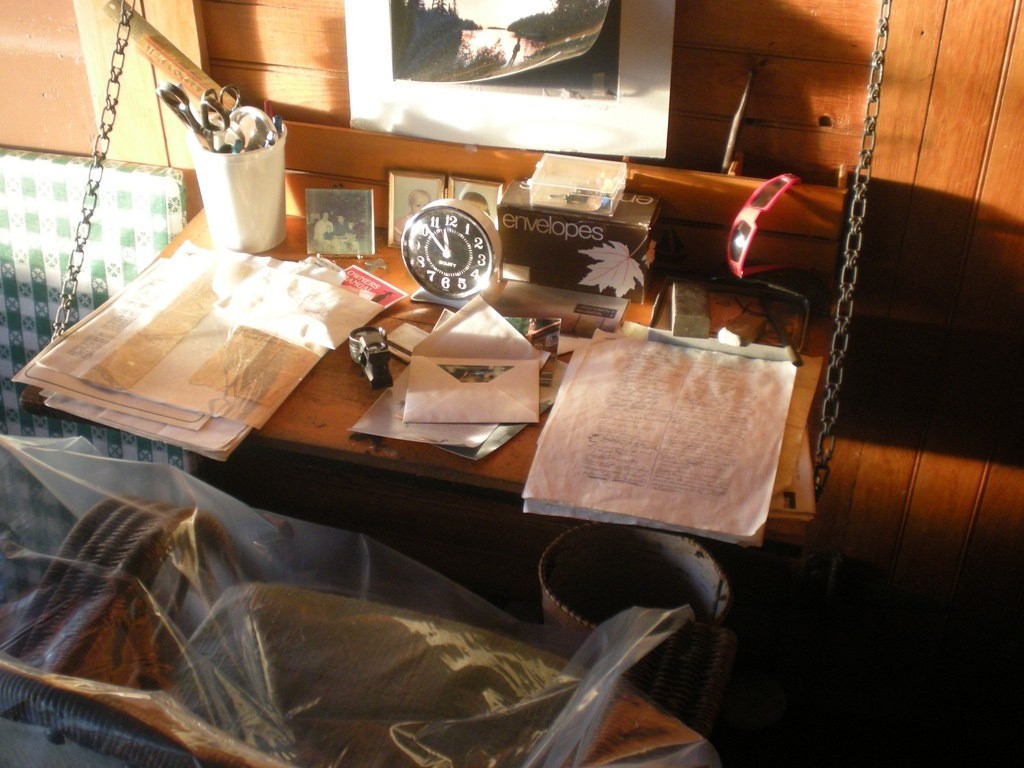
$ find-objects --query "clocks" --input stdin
[399,198,501,312]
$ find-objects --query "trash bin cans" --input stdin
[533,517,734,706]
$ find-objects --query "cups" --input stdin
[182,119,287,254]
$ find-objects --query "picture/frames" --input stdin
[305,188,375,256]
[448,175,503,229]
[388,170,446,248]
[345,0,676,163]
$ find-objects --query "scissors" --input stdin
[152,76,245,153]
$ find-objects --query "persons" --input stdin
[505,36,521,69]
[395,189,431,244]
[314,210,360,255]
[462,191,491,219]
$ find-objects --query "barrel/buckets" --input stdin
[537,521,733,634]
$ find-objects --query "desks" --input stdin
[36,206,844,556]
